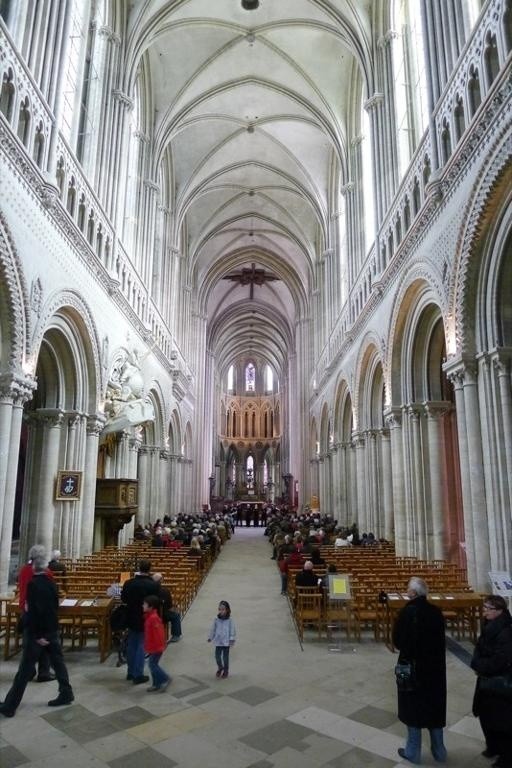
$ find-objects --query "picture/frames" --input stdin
[56,470,82,501]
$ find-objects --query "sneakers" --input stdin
[0,702,14,717]
[171,635,183,642]
[398,747,406,758]
[128,675,172,694]
[217,669,228,678]
[37,673,57,682]
[482,750,506,767]
[47,693,74,706]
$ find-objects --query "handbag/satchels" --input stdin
[394,665,416,688]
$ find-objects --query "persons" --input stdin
[152,572,183,642]
[134,502,379,586]
[0,557,74,717]
[391,576,446,765]
[141,595,172,693]
[206,600,237,679]
[19,544,57,682]
[120,559,162,684]
[470,595,512,767]
[48,550,67,589]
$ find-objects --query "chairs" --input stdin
[0,539,215,650]
[274,533,487,643]
